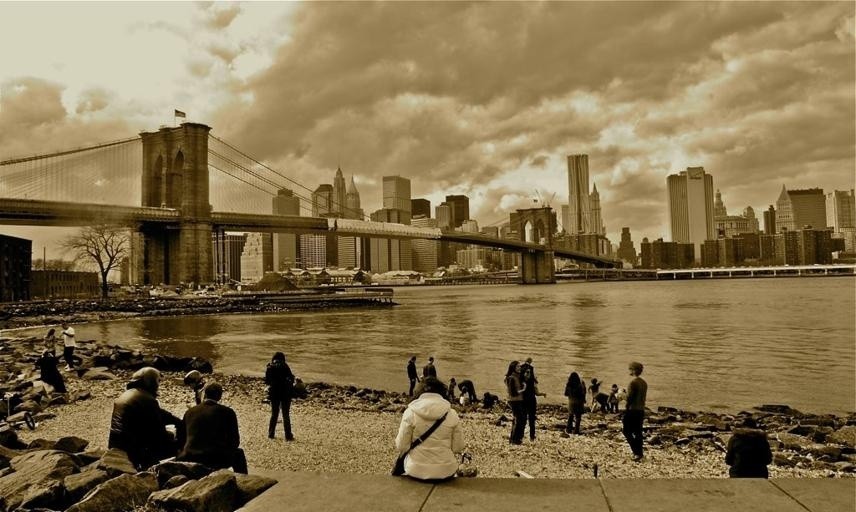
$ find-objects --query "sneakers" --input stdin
[566,430,580,435]
[589,408,620,415]
[268,431,275,438]
[508,433,524,445]
[63,363,75,373]
[285,430,294,442]
[631,452,644,462]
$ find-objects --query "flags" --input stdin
[174,110,185,117]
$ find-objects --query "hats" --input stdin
[123,365,162,385]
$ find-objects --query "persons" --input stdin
[34,350,67,395]
[579,378,626,414]
[423,356,437,376]
[446,377,457,401]
[504,360,526,445]
[724,419,772,480]
[482,390,497,409]
[407,357,418,395]
[456,379,478,401]
[518,366,547,439]
[105,365,181,465]
[43,328,56,352]
[176,384,247,472]
[395,377,464,482]
[59,321,76,371]
[564,372,587,434]
[263,351,294,440]
[521,356,539,385]
[615,361,647,461]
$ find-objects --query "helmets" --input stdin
[184,370,203,386]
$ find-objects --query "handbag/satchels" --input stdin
[390,443,412,477]
